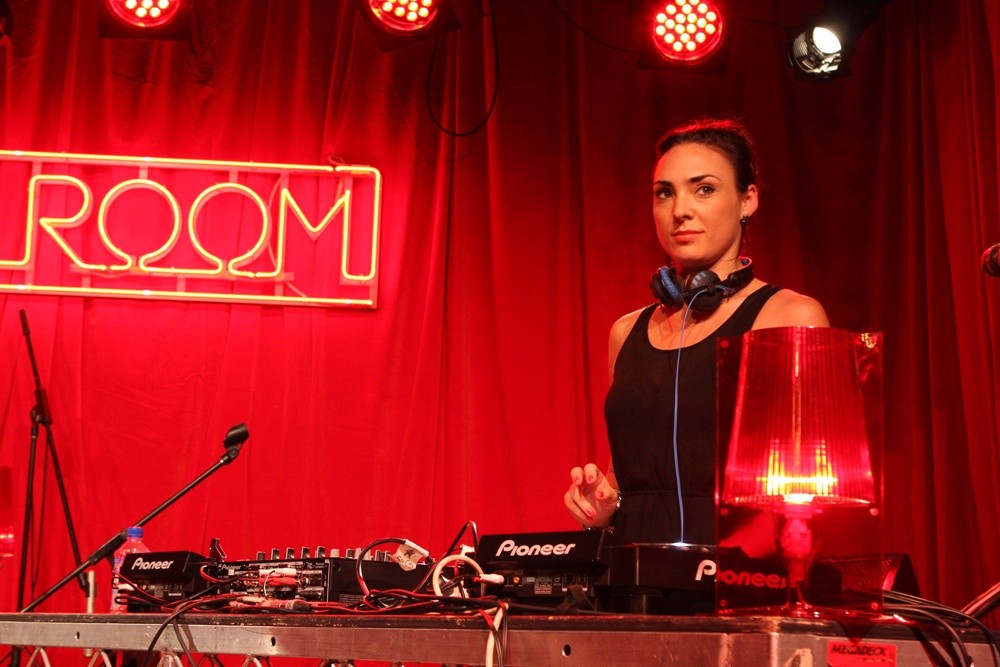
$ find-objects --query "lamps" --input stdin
[95,0,193,41]
[354,0,463,52]
[788,0,882,81]
[720,326,876,618]
[648,0,729,66]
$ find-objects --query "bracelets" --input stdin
[581,489,623,532]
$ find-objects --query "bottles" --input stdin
[110,525,151,613]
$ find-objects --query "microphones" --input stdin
[648,255,754,313]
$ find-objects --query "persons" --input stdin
[563,120,830,557]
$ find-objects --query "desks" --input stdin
[0,613,1000,667]
